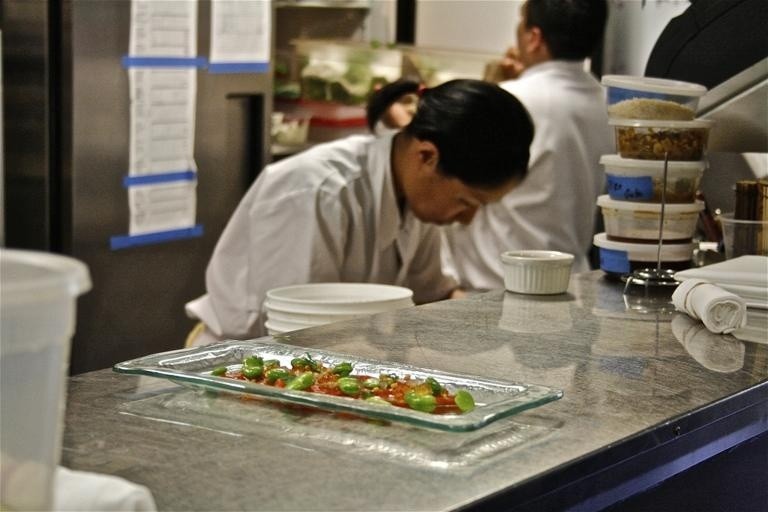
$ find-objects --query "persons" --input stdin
[183,79,534,351]
[643,0,768,94]
[436,0,609,294]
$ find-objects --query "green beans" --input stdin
[212,355,475,412]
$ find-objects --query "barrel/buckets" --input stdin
[262,282,415,337]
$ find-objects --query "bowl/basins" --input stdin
[499,251,573,296]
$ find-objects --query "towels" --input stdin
[670,254,768,335]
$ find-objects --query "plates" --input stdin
[116,388,564,476]
[114,337,564,434]
